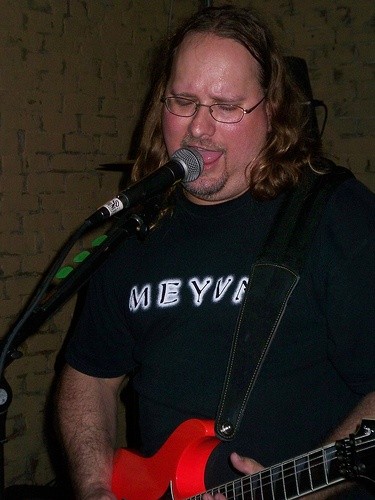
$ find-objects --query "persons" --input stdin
[57,6,375,500]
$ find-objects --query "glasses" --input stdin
[161,93,268,125]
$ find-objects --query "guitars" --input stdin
[84,415,375,499]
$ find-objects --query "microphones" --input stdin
[87,147,204,226]
